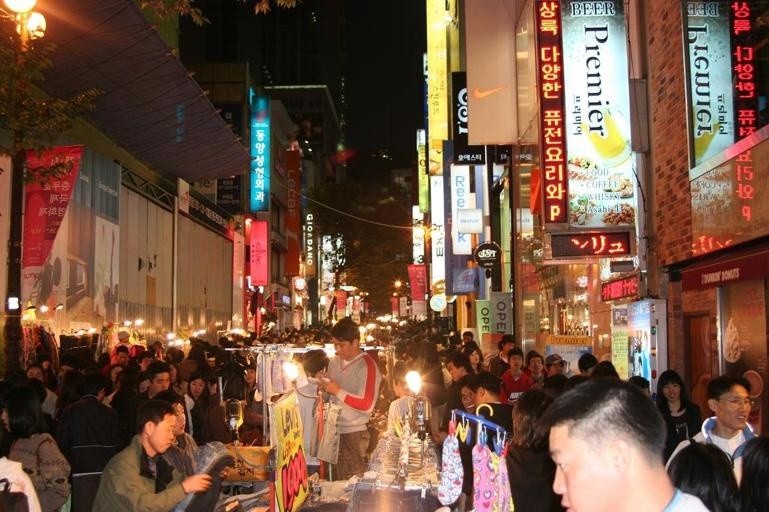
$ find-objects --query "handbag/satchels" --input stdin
[35,438,72,512]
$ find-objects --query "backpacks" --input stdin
[0,478,30,512]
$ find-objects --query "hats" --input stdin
[545,353,566,367]
